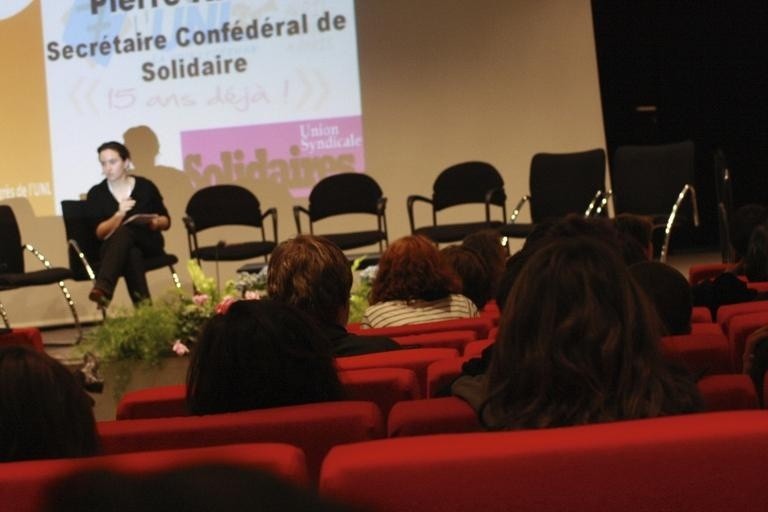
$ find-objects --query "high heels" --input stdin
[88,289,106,310]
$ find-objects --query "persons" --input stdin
[1,337,100,460]
[69,348,104,393]
[87,141,171,311]
[547,212,766,379]
[266,234,406,358]
[360,224,555,330]
[452,240,703,434]
[188,297,347,416]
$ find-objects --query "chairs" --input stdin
[404,160,510,252]
[1,203,86,348]
[181,183,280,274]
[651,140,734,263]
[60,199,185,326]
[0,261,768,511]
[295,171,390,266]
[495,146,612,240]
